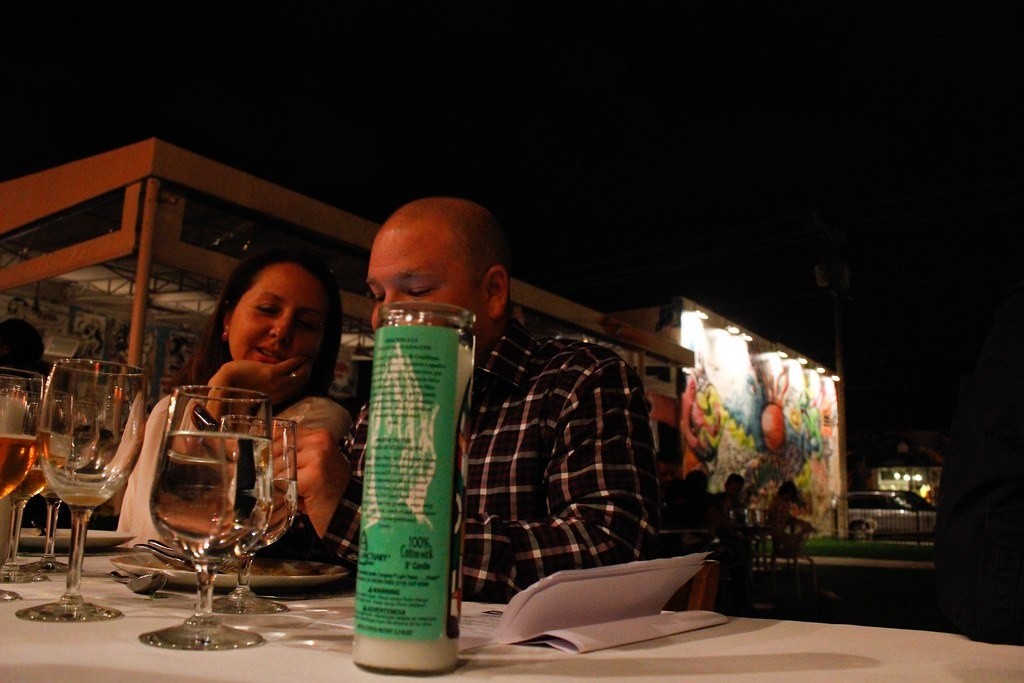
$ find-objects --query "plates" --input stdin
[19,528,137,549]
[109,553,350,593]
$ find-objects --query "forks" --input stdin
[148,539,239,573]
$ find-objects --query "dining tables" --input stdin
[0,555,1024,682]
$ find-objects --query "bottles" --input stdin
[351,302,476,672]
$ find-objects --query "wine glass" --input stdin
[193,414,298,616]
[139,385,274,652]
[0,359,146,624]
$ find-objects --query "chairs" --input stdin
[767,517,818,605]
[684,560,721,612]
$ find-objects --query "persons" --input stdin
[0,318,73,551]
[768,482,799,567]
[258,197,664,603]
[935,292,1024,645]
[116,247,349,551]
[666,472,753,594]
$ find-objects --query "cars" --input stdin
[848,490,936,544]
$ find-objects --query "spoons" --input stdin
[110,570,167,596]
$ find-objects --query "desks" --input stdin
[718,526,777,603]
